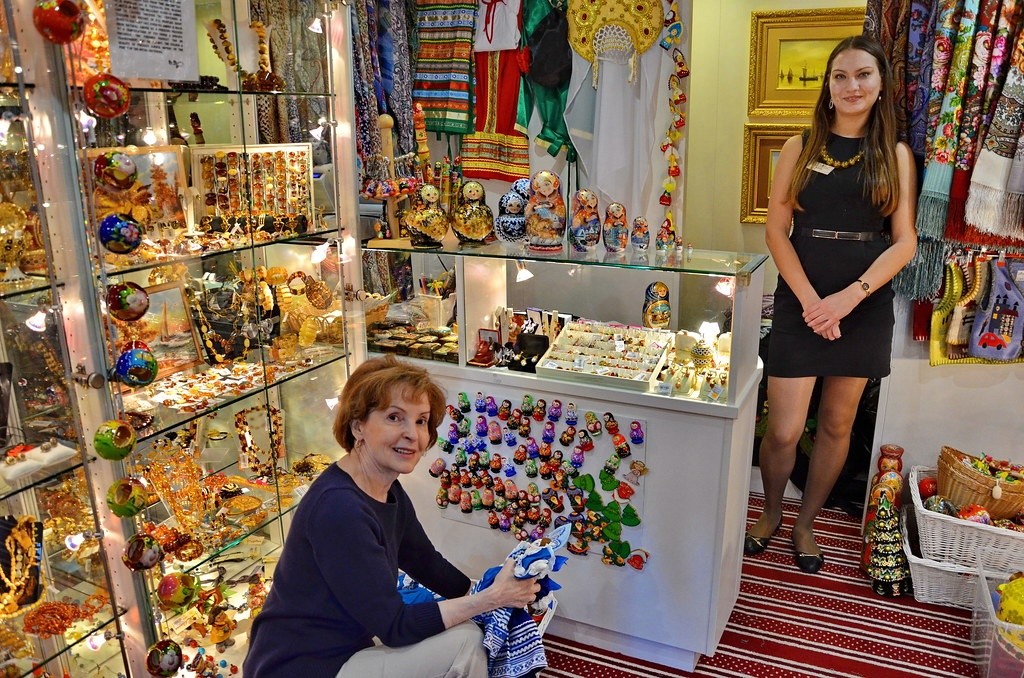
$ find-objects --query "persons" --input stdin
[243,352,541,678]
[744,36,919,574]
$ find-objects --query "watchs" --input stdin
[857,279,869,297]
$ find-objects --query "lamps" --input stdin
[515,259,534,283]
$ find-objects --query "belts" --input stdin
[791,226,885,243]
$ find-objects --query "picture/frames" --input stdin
[747,6,865,124]
[740,123,818,225]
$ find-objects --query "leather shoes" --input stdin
[790,527,824,573]
[743,511,783,555]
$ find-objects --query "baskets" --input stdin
[899,505,1024,613]
[909,465,1024,575]
[937,445,1024,520]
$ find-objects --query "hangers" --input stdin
[948,244,1024,270]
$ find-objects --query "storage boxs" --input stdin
[371,306,676,390]
[972,545,1024,678]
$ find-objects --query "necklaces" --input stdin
[1,0,348,677]
[821,141,865,167]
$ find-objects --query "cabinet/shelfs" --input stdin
[0,1,367,678]
[361,235,770,674]
